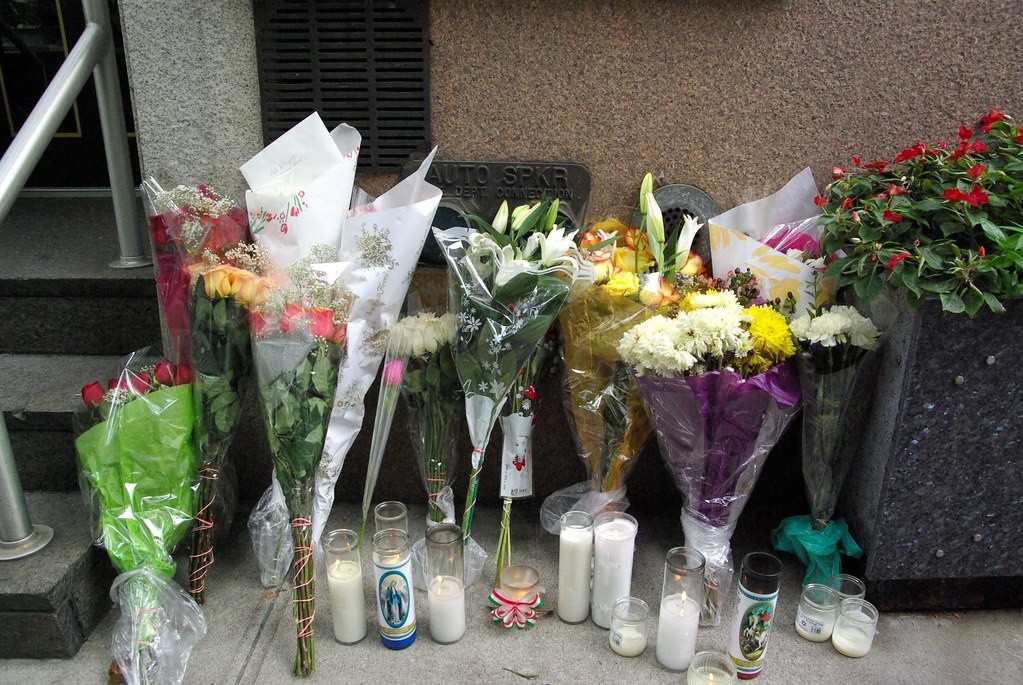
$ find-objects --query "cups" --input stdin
[609,598,650,658]
[795,574,880,658]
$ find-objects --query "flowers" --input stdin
[75,110,1023,685]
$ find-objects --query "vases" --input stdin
[842,269,1023,602]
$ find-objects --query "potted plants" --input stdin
[656,592,699,670]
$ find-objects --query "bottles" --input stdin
[558,511,638,630]
[656,547,706,672]
[322,500,465,649]
[726,552,784,680]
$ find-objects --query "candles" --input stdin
[591,522,637,628]
[373,552,417,650]
[688,673,732,685]
[833,609,876,658]
[559,525,590,621]
[796,612,836,642]
[327,560,367,642]
[430,575,465,642]
[611,629,647,657]
